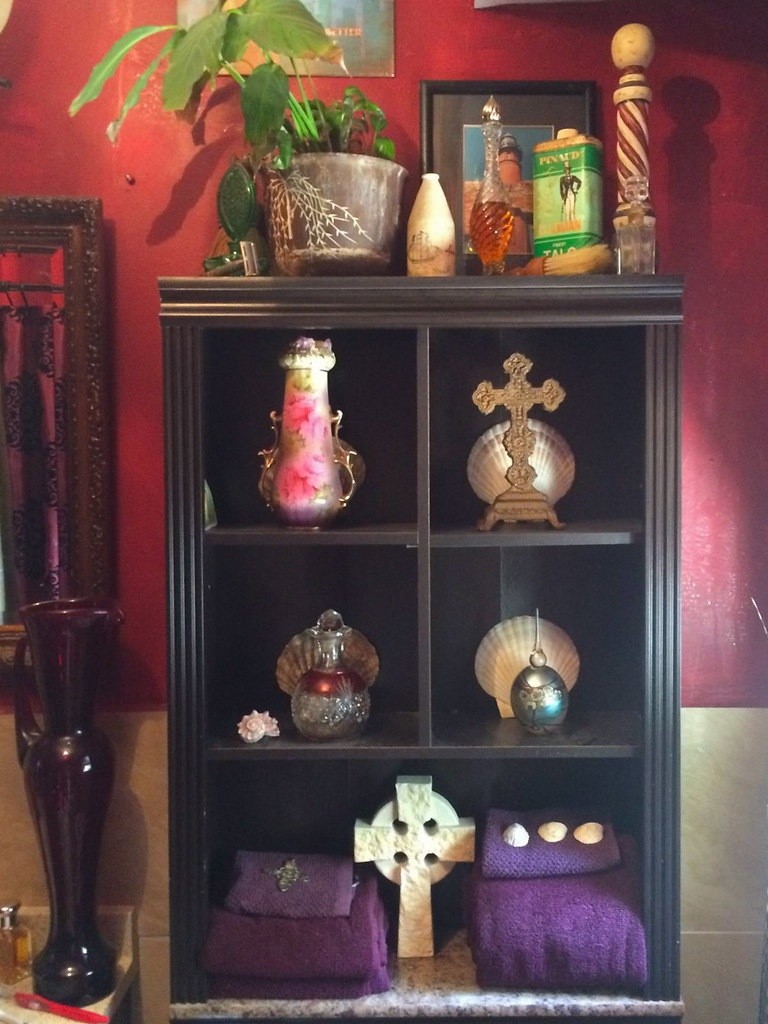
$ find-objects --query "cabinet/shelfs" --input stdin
[156,273,688,1024]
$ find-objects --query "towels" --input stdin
[199,848,388,999]
[467,806,647,992]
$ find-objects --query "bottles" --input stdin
[469,94,515,276]
[407,173,455,277]
[13,598,119,1005]
[292,610,371,744]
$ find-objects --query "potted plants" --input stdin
[69,1,408,275]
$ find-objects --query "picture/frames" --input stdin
[417,77,598,276]
[0,195,119,675]
